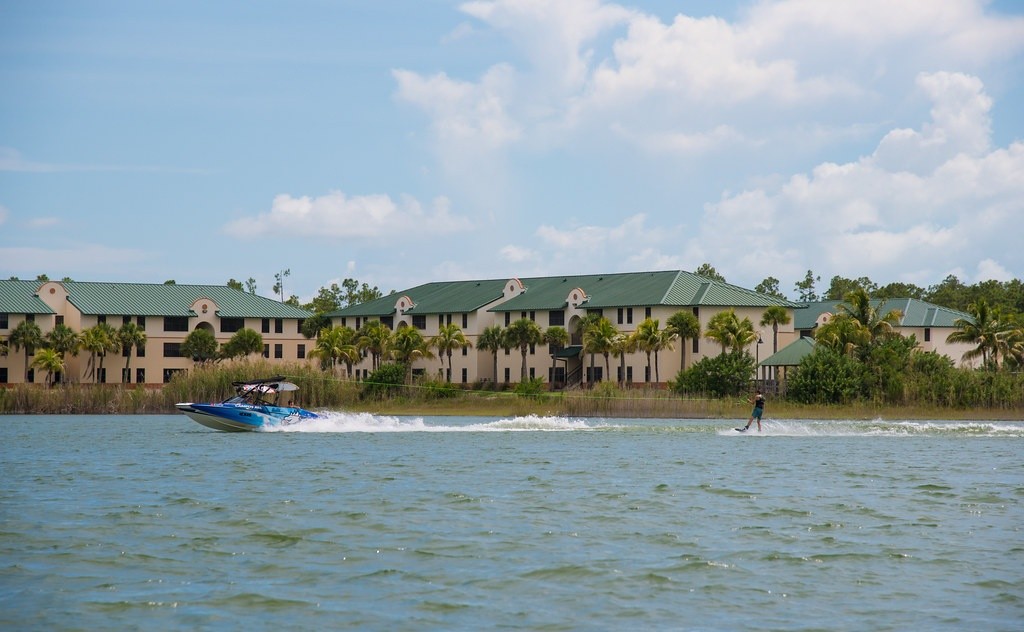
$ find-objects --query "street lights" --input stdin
[756,334,763,391]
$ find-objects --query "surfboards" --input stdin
[735,428,745,432]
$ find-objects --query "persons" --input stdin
[287,400,302,409]
[739,391,766,432]
[238,388,255,405]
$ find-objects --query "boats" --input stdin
[174,376,319,430]
